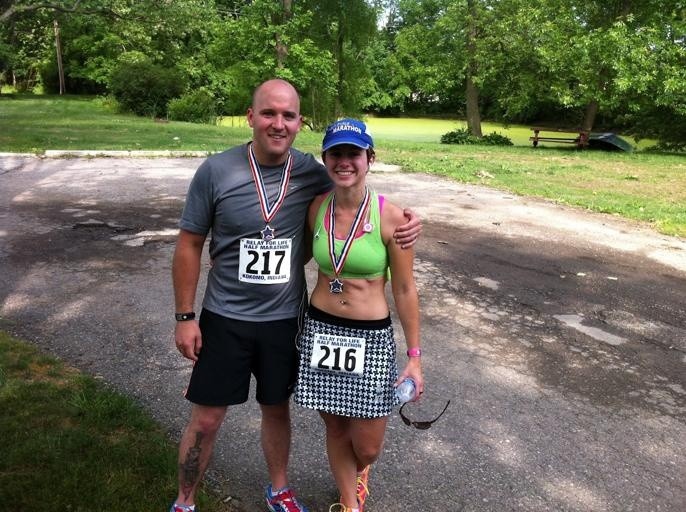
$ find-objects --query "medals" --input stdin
[260,225,275,239]
[328,279,344,294]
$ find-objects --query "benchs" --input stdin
[529,127,590,149]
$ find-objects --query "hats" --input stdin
[321,118,375,152]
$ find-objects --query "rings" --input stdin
[419,391,423,395]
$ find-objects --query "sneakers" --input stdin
[170,501,196,512]
[265,481,310,512]
[339,464,370,512]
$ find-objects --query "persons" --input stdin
[166,77,424,511]
[302,116,426,512]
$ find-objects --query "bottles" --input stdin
[394,378,416,403]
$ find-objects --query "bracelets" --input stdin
[174,312,196,321]
[407,348,422,357]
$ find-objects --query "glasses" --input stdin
[398,399,450,430]
